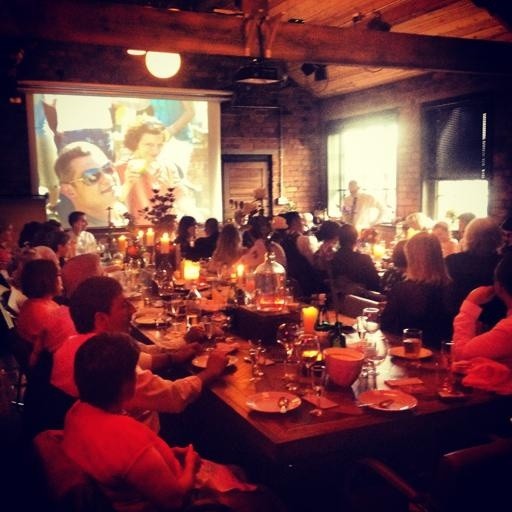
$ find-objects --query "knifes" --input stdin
[358,398,393,408]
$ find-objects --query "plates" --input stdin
[388,345,433,360]
[359,388,418,412]
[247,390,302,414]
[192,355,238,369]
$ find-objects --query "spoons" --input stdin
[277,397,290,413]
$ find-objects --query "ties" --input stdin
[351,197,357,217]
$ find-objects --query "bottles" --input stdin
[364,360,377,391]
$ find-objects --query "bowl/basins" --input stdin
[323,347,366,389]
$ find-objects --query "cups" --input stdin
[97,243,328,351]
[313,210,323,224]
[360,243,368,255]
[402,328,423,356]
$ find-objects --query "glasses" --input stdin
[68,162,115,187]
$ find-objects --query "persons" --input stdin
[339,180,382,228]
[44,95,117,162]
[51,141,132,228]
[113,120,204,224]
[0,210,510,512]
[135,99,196,144]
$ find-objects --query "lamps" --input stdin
[232,66,280,86]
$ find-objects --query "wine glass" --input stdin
[356,306,387,377]
[310,365,328,416]
[249,323,320,392]
[440,338,457,396]
